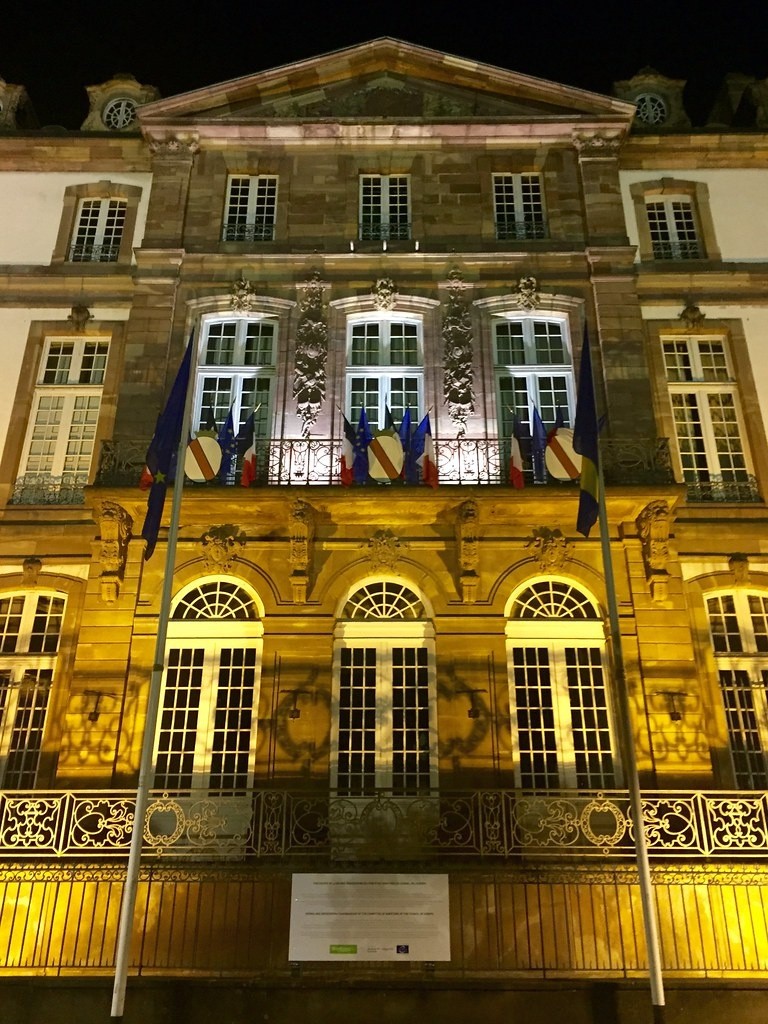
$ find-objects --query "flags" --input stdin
[207,408,218,431]
[555,408,564,429]
[509,412,525,488]
[218,410,235,483]
[533,406,547,483]
[572,319,600,539]
[598,414,607,433]
[238,411,257,486]
[339,404,438,489]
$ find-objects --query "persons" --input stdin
[141,326,197,562]
